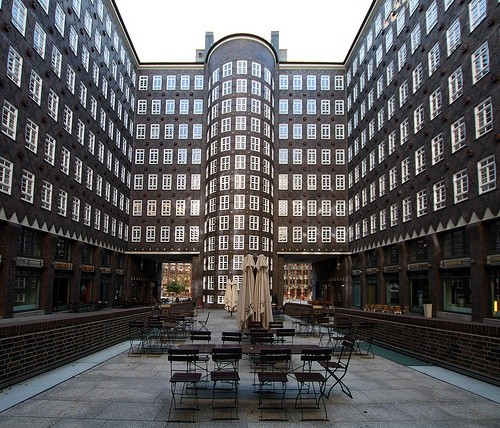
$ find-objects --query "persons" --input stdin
[152,295,191,313]
[293,294,305,301]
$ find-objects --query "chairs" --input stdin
[126,302,376,423]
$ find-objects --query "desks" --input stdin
[179,344,318,387]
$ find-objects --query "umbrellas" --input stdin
[236,254,273,330]
[224,279,238,317]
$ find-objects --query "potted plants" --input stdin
[422,299,432,319]
